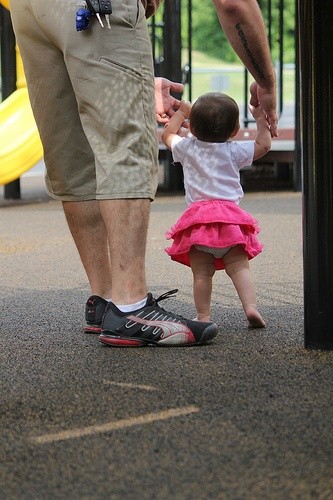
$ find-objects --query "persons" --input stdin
[8,0,279,347]
[161,92,272,327]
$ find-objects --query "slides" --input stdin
[2,85,47,186]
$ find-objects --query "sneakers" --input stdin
[99,289,219,348]
[85,293,106,332]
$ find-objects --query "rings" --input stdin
[162,113,166,118]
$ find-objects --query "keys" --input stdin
[76,0,112,31]
